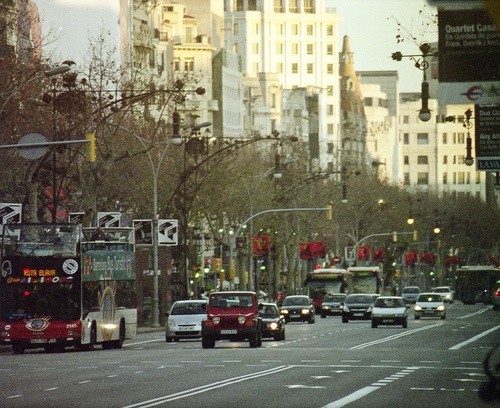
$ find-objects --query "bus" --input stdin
[454,265,500,305]
[305,265,386,316]
[0,223,138,353]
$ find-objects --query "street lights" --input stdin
[93,118,183,328]
[226,157,296,292]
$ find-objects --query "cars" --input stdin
[163,299,208,342]
[370,296,411,329]
[490,280,500,310]
[247,302,286,341]
[372,294,381,304]
[413,293,447,320]
[430,286,456,303]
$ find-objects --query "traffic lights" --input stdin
[85,134,97,163]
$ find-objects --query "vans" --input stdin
[400,286,424,305]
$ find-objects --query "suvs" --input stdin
[340,294,373,323]
[278,295,315,324]
[201,291,264,349]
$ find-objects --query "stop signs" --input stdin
[333,257,341,265]
